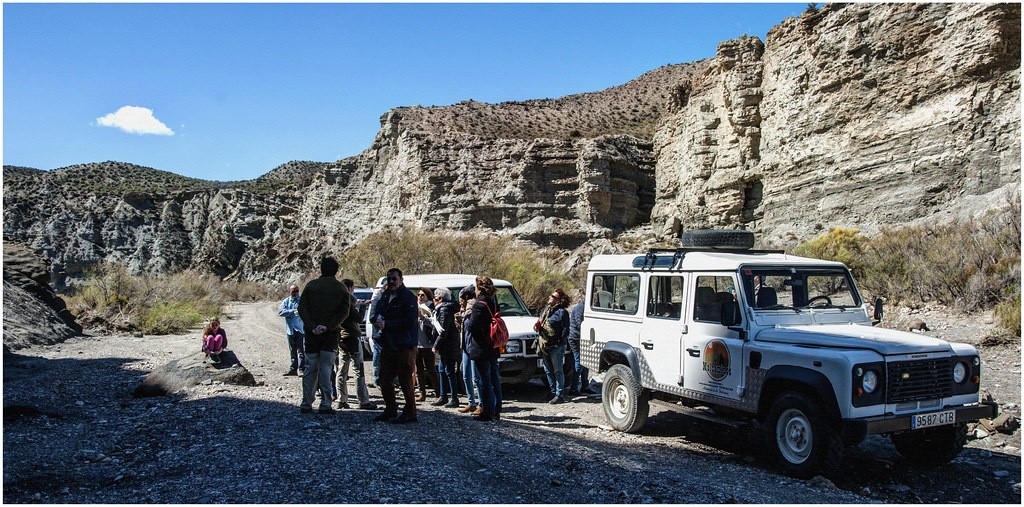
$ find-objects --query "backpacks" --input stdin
[479,300,510,352]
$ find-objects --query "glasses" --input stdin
[418,294,425,296]
[550,294,558,301]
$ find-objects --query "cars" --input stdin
[353,289,375,360]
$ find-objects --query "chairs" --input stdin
[655,287,781,322]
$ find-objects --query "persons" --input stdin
[732,275,766,308]
[459,276,502,421]
[366,276,401,394]
[568,290,602,397]
[202,317,228,363]
[279,285,305,377]
[454,284,496,415]
[336,279,378,410]
[297,258,349,414]
[415,287,441,402]
[533,288,570,404]
[374,268,419,423]
[430,286,460,407]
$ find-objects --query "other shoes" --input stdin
[494,411,501,420]
[283,370,297,376]
[580,387,596,394]
[474,413,493,421]
[570,388,582,396]
[212,356,222,363]
[361,402,377,410]
[337,402,350,409]
[540,395,554,402]
[320,408,335,415]
[209,356,215,364]
[548,394,563,404]
[298,369,304,377]
[302,406,313,413]
[416,393,426,402]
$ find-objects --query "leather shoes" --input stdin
[445,398,459,408]
[471,409,482,416]
[458,405,476,413]
[431,397,448,406]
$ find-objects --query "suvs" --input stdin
[364,273,551,396]
[579,229,999,480]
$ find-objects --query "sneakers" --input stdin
[393,412,418,424]
[376,411,397,420]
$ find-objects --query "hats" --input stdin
[459,284,476,300]
